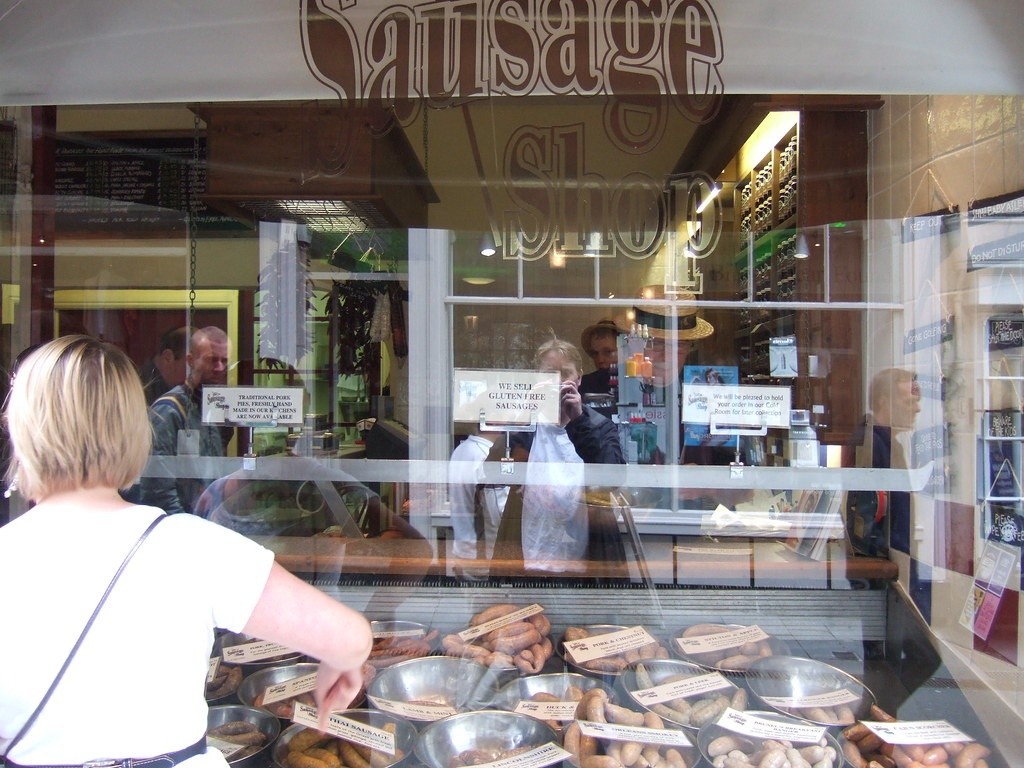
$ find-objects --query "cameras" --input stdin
[559,385,572,401]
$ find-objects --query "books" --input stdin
[777,484,843,561]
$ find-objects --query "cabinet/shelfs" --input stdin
[734,109,869,444]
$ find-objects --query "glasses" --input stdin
[643,337,693,354]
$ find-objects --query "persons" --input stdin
[451,339,631,583]
[579,286,752,512]
[840,370,941,722]
[192,358,434,660]
[698,370,732,445]
[0,334,373,768]
[138,326,212,408]
[140,326,231,514]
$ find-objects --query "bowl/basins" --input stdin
[697,710,845,768]
[441,624,555,674]
[212,631,320,678]
[556,721,702,768]
[745,654,878,739]
[485,672,620,745]
[413,710,562,768]
[367,656,500,733]
[572,489,662,536]
[271,709,417,768]
[669,625,791,672]
[620,658,751,733]
[207,661,244,707]
[837,718,909,768]
[370,620,443,655]
[555,626,672,675]
[209,704,281,768]
[236,663,366,731]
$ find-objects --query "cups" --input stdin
[634,353,644,376]
[643,392,652,407]
[651,392,656,405]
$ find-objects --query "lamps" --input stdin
[547,238,567,270]
[795,231,810,259]
[478,227,497,257]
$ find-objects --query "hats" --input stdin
[580,316,632,358]
[612,285,714,341]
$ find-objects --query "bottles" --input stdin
[641,357,652,376]
[625,356,636,376]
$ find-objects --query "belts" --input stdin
[4,733,207,768]
